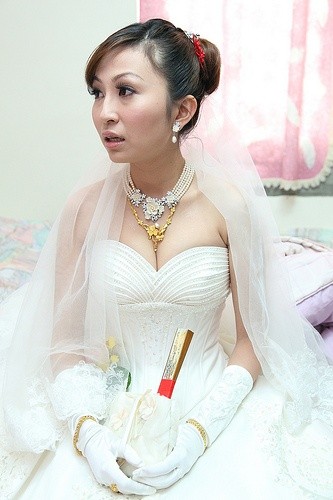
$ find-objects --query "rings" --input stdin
[110,482,119,493]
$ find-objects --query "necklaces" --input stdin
[121,161,195,252]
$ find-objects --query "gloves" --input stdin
[130,366,254,489]
[48,379,155,495]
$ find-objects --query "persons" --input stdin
[0,17,333,500]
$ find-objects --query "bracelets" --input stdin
[72,415,97,457]
[185,418,208,448]
